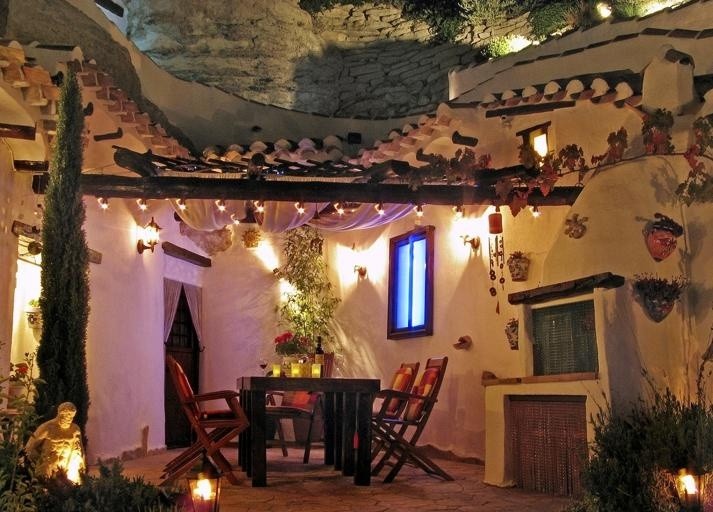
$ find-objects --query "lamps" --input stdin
[185,448,222,511]
[671,449,707,511]
[136,216,162,254]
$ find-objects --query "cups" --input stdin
[290,363,300,378]
[272,364,280,377]
[311,363,321,378]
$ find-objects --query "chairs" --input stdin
[160,352,248,485]
[370,356,454,484]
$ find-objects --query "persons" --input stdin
[26,401,86,485]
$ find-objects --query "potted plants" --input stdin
[269,224,341,376]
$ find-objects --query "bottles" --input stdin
[314,336,325,378]
